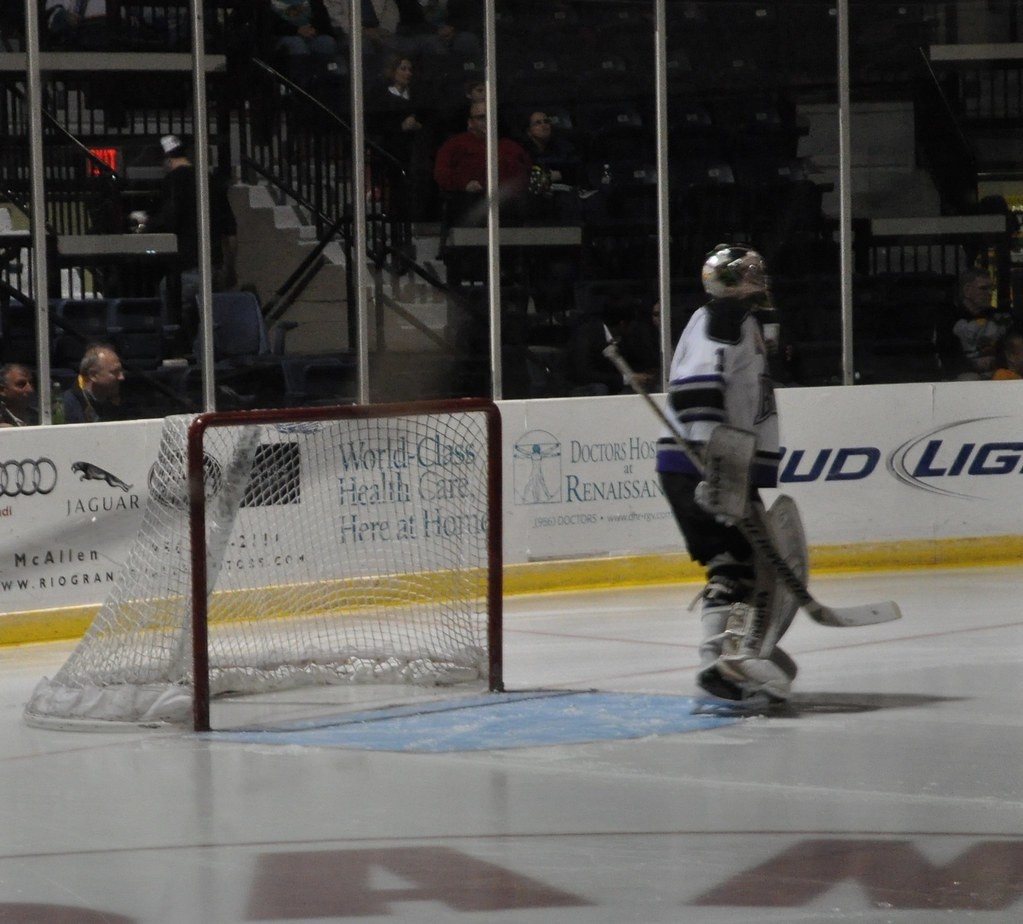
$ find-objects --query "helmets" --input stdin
[700,243,769,297]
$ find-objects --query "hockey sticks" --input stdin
[602,344,903,627]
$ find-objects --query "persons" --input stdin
[0,362,39,427]
[363,54,443,156]
[134,133,239,272]
[656,245,804,708]
[932,267,1023,380]
[60,341,126,421]
[434,94,528,192]
[43,0,519,177]
[521,108,565,170]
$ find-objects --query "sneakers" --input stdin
[690,669,769,720]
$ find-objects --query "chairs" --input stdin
[44,295,360,413]
